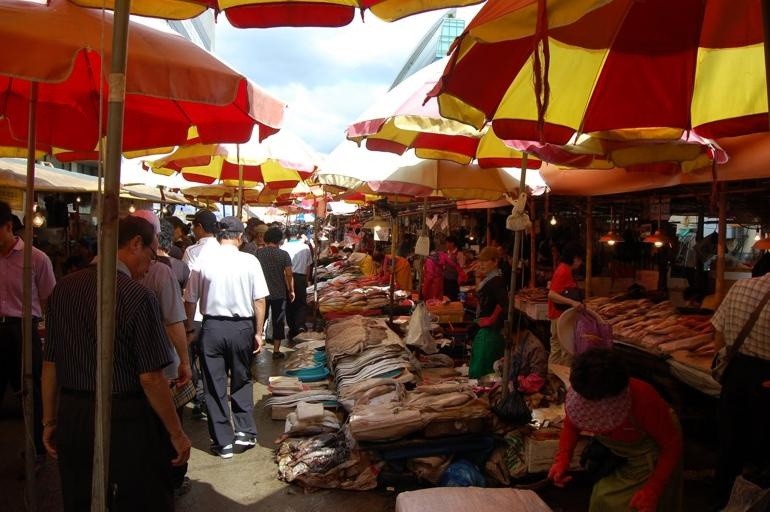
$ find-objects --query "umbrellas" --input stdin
[180,183,293,218]
[141,142,318,219]
[346,50,729,399]
[313,132,550,296]
[1,1,285,511]
[421,0,769,157]
[47,0,482,508]
[331,192,386,210]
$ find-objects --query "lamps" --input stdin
[599,203,623,246]
[643,204,673,248]
[33,202,44,227]
[161,203,167,214]
[363,216,392,229]
[549,215,557,225]
[128,200,136,214]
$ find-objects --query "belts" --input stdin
[0,316,42,322]
[61,387,147,400]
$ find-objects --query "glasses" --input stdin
[149,247,157,264]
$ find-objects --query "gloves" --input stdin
[547,431,577,488]
[627,456,678,512]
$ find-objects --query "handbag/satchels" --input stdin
[710,345,730,384]
[553,287,582,310]
[575,313,613,353]
[168,377,196,410]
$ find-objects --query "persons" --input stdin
[710,254,769,512]
[483,309,547,398]
[337,234,467,310]
[548,345,684,510]
[548,247,584,365]
[344,227,365,252]
[469,238,513,380]
[1,201,316,511]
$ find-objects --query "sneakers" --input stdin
[210,443,233,458]
[273,352,284,360]
[234,431,256,446]
[174,477,192,501]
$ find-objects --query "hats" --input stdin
[186,208,216,225]
[474,246,498,260]
[565,381,633,430]
[216,216,247,232]
